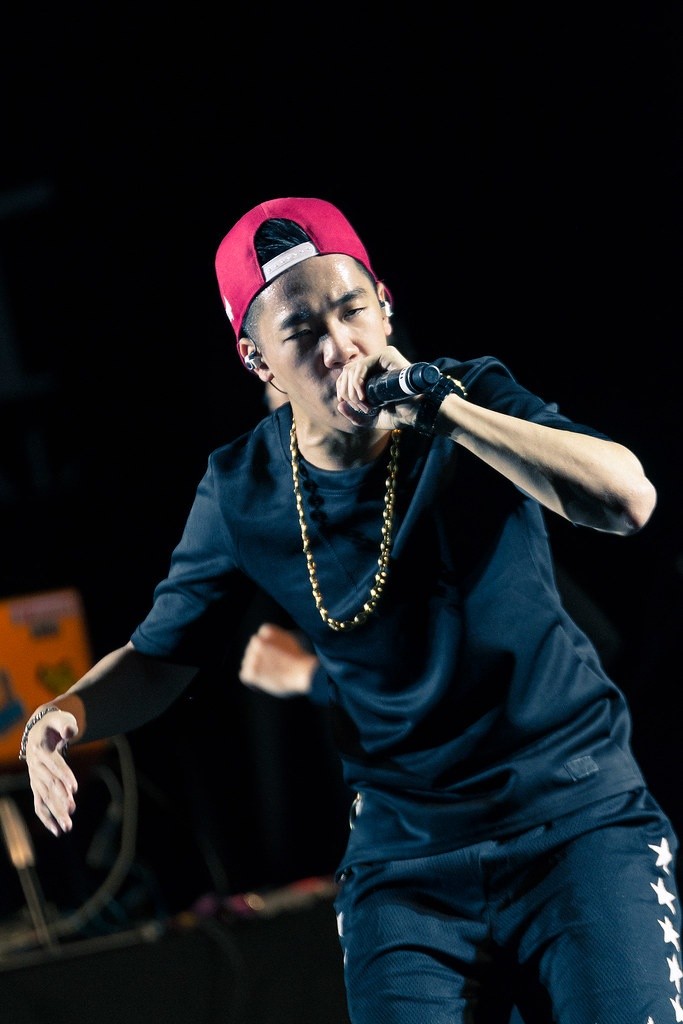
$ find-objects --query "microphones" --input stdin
[355,362,440,417]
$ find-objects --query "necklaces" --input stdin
[289,415,406,635]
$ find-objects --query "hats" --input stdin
[215,197,392,368]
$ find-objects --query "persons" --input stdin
[239,621,344,706]
[24,195,683,1024]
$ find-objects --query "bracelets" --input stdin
[17,706,60,763]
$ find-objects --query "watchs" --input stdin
[413,372,468,438]
